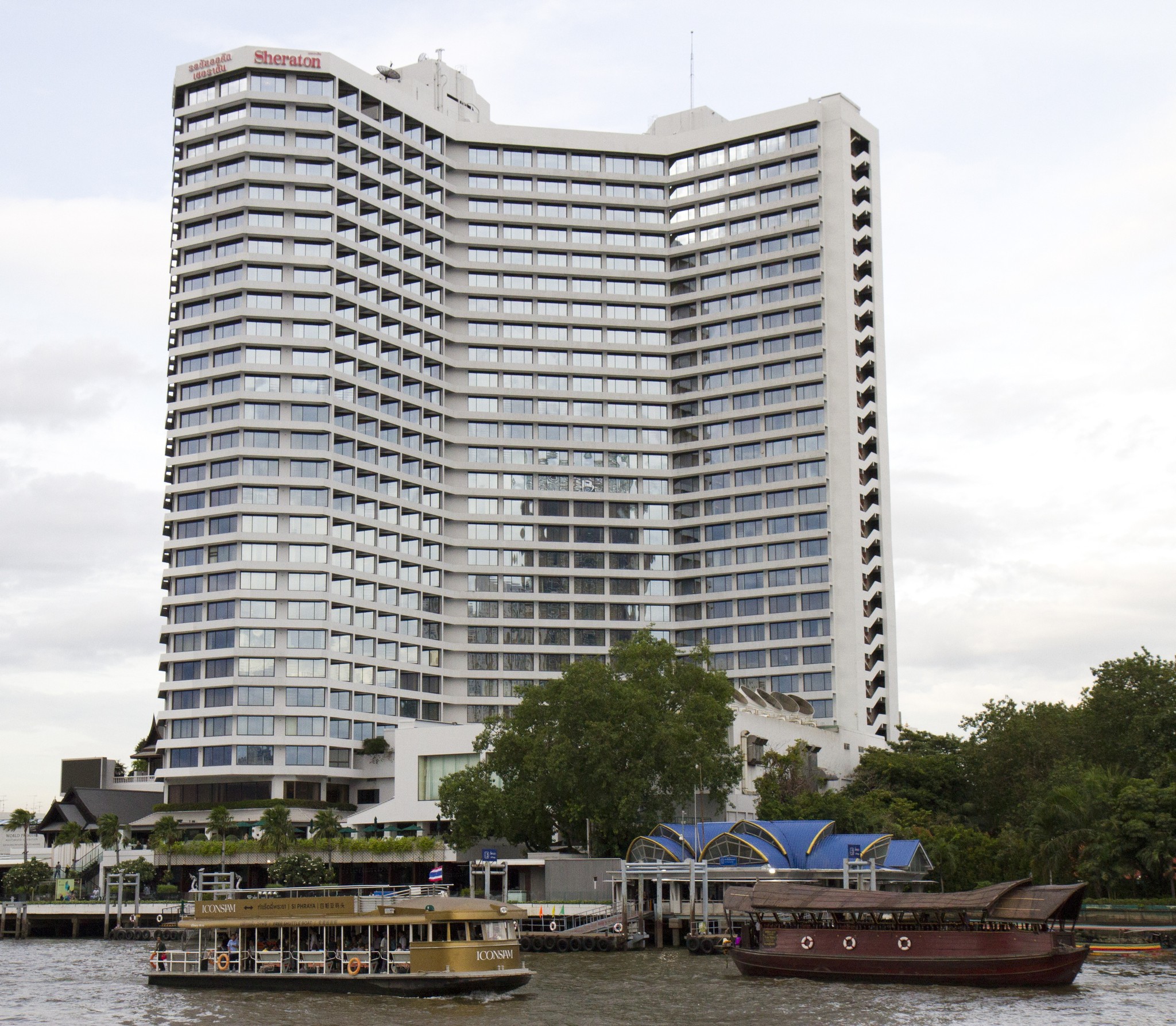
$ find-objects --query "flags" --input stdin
[428,866,443,882]
[539,905,564,919]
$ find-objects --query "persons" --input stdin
[462,922,481,940]
[220,925,411,974]
[633,889,639,911]
[434,929,447,941]
[144,884,151,896]
[643,891,648,911]
[60,892,76,902]
[750,920,760,948]
[154,936,166,972]
[54,861,61,879]
[244,833,248,841]
[92,886,100,900]
[64,865,71,879]
[135,839,150,850]
[711,895,716,900]
[369,893,374,896]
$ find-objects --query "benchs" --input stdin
[259,952,289,973]
[302,953,333,973]
[345,952,378,973]
[391,953,411,968]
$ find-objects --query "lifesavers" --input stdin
[550,922,556,931]
[206,950,214,964]
[801,936,813,949]
[151,953,157,969]
[108,929,187,941]
[700,938,715,953]
[614,923,623,932]
[513,921,517,930]
[130,914,136,922]
[898,937,911,950]
[843,936,856,950]
[347,957,361,976]
[685,936,700,952]
[519,936,612,953]
[156,915,163,922]
[217,953,230,970]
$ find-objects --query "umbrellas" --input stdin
[290,827,305,838]
[338,827,359,837]
[236,819,287,841]
[310,818,313,838]
[180,898,185,920]
[363,824,425,838]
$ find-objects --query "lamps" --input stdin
[499,907,508,914]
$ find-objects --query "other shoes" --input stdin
[380,971,387,974]
[235,970,239,973]
[229,970,232,972]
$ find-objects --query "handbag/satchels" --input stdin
[161,952,166,960]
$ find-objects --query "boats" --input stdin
[142,884,537,997]
[713,877,1091,985]
